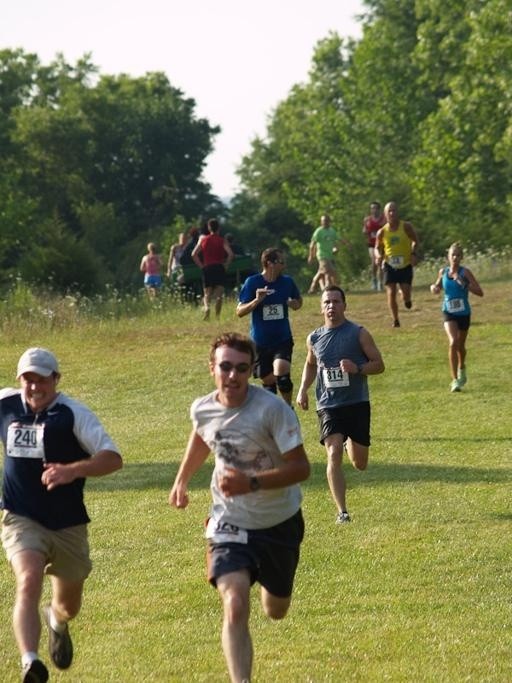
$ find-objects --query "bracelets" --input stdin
[462,283,468,289]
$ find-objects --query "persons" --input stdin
[0,348,122,683]
[168,331,310,683]
[307,201,420,327]
[431,243,484,392]
[296,287,384,523]
[140,219,244,320]
[236,248,302,410]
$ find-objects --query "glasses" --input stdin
[219,360,250,372]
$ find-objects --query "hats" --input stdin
[16,346,59,380]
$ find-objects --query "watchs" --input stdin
[358,364,363,374]
[249,473,260,492]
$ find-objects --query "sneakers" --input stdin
[21,659,48,682]
[42,603,73,669]
[458,366,467,386]
[335,511,351,525]
[405,301,412,308]
[450,378,461,393]
[392,319,400,326]
